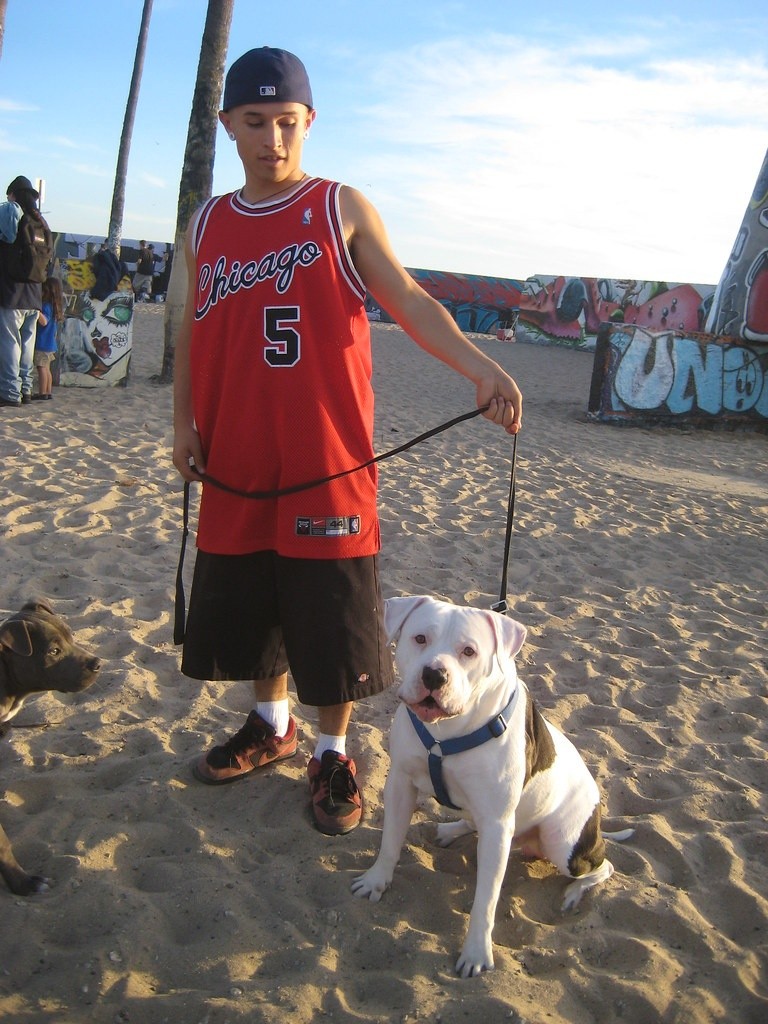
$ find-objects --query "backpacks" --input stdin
[5,212,53,283]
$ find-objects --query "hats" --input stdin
[223,45,313,112]
[6,176,39,199]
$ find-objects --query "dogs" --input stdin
[350,595,637,982]
[0,600,104,901]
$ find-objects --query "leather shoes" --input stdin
[31,394,53,400]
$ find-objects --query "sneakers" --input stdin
[308,749,361,836]
[0,397,21,407]
[192,709,297,785]
[21,394,31,404]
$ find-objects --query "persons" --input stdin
[31,277,64,399]
[0,176,42,407]
[169,46,524,838]
[101,236,174,303]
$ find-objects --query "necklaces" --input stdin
[249,173,308,203]
